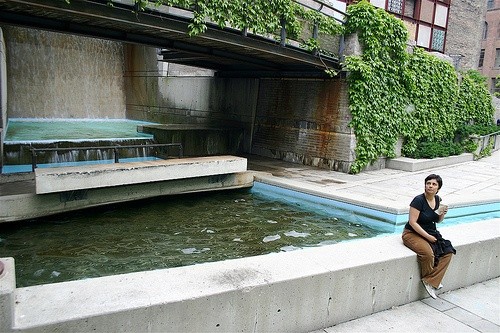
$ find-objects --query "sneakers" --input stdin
[436,282,443,289]
[422,279,437,300]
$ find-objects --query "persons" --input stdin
[402,174,453,300]
